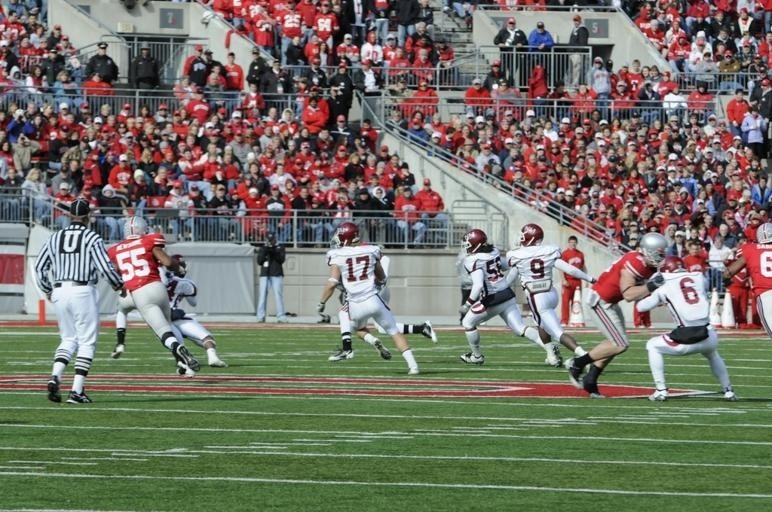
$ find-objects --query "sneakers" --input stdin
[460,353,484,364]
[545,343,605,400]
[66,391,92,403]
[649,389,668,401]
[208,356,228,367]
[329,321,437,375]
[48,376,61,402]
[111,344,124,358]
[173,342,200,376]
[724,391,739,401]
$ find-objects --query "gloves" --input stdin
[647,273,665,291]
[458,304,469,313]
[316,302,324,311]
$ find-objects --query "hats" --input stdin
[343,31,394,41]
[312,57,371,68]
[194,45,213,55]
[71,200,89,218]
[432,110,743,235]
[60,102,431,191]
[474,59,507,85]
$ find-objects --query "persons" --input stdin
[635,255,737,401]
[560,235,589,328]
[107,215,200,376]
[385,0,772,330]
[317,222,438,376]
[455,229,563,368]
[723,223,771,335]
[255,232,289,323]
[35,198,127,404]
[506,223,597,373]
[0,0,454,250]
[111,232,228,368]
[565,232,668,399]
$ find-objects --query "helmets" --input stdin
[640,232,688,273]
[757,223,772,244]
[461,229,487,254]
[330,223,360,249]
[124,216,148,239]
[519,224,543,246]
